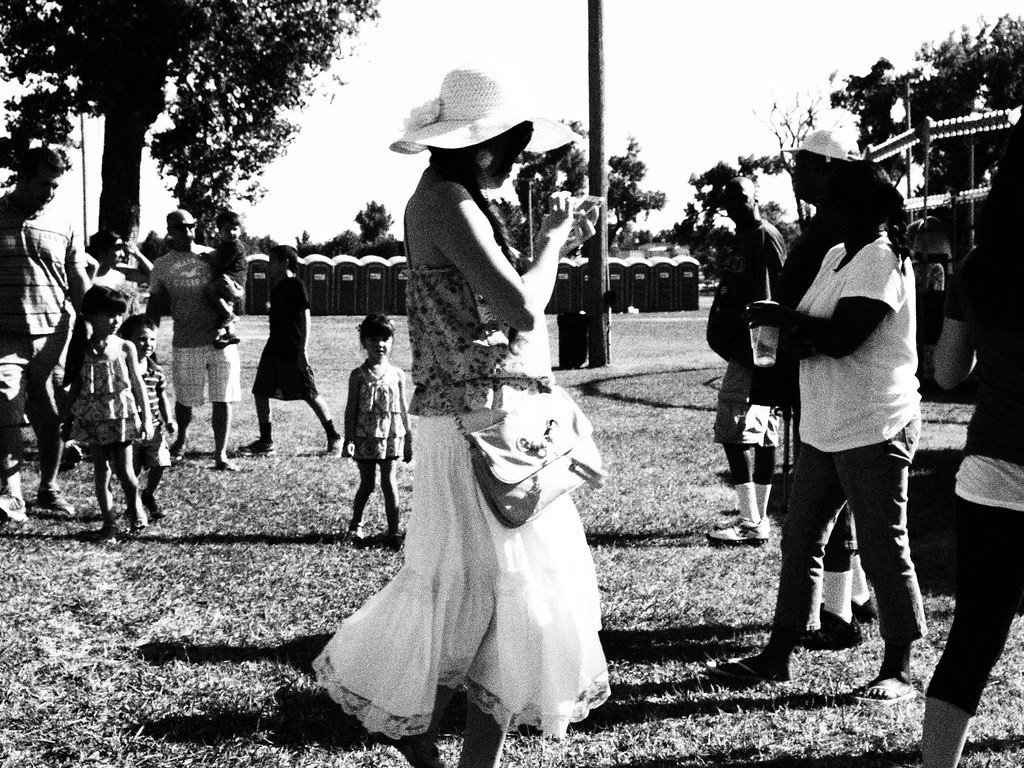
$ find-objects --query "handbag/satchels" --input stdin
[463,389,609,527]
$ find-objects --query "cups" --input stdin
[746,300,779,367]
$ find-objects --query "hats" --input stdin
[81,285,131,315]
[782,127,863,164]
[388,69,577,154]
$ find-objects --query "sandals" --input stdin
[172,436,191,462]
[218,459,241,473]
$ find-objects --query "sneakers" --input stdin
[238,438,277,455]
[322,434,346,460]
[714,516,750,530]
[706,516,769,545]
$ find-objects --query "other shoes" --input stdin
[219,313,239,328]
[348,516,365,541]
[37,487,76,516]
[799,605,864,650]
[0,485,28,521]
[385,515,404,541]
[100,513,118,540]
[374,726,444,768]
[129,505,147,534]
[851,601,878,623]
[141,489,167,517]
[213,334,240,350]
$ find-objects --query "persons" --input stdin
[314,67,614,768]
[198,210,247,347]
[62,285,153,539]
[342,312,412,546]
[145,210,242,473]
[923,140,1024,768]
[89,232,153,324]
[707,177,790,545]
[706,129,928,703]
[237,245,344,460]
[119,314,179,518]
[0,146,91,522]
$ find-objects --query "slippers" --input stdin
[854,684,919,705]
[706,661,794,683]
